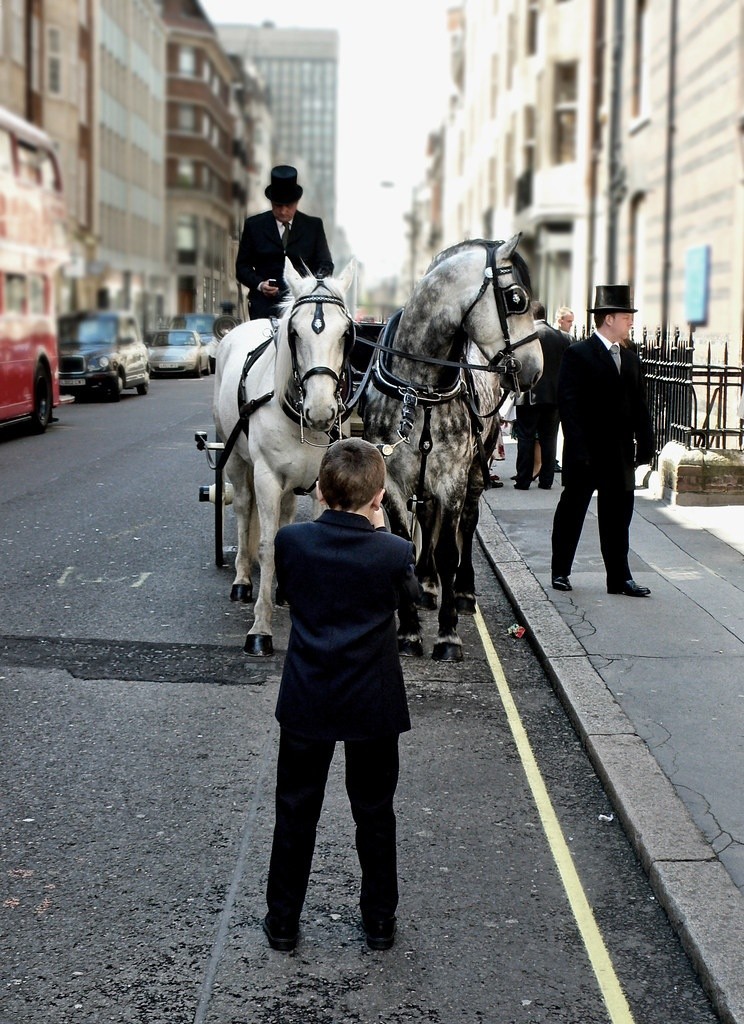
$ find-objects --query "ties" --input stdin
[282,222,293,250]
[610,344,622,375]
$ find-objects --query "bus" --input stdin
[0,108,68,435]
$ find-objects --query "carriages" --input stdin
[194,233,544,664]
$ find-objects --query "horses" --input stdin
[211,255,356,658]
[362,230,545,663]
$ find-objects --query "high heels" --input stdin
[511,468,540,481]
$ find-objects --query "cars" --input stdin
[145,329,211,380]
[170,314,222,375]
[57,311,153,403]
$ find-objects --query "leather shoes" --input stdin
[263,912,299,951]
[364,915,397,949]
[551,571,572,591]
[607,578,651,597]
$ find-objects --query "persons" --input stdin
[491,285,656,597]
[236,165,334,321]
[261,437,416,953]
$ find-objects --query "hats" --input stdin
[264,165,304,203]
[586,284,639,313]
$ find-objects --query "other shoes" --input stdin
[555,459,562,472]
[489,480,503,488]
[513,484,530,490]
[489,468,500,480]
[537,483,552,490]
